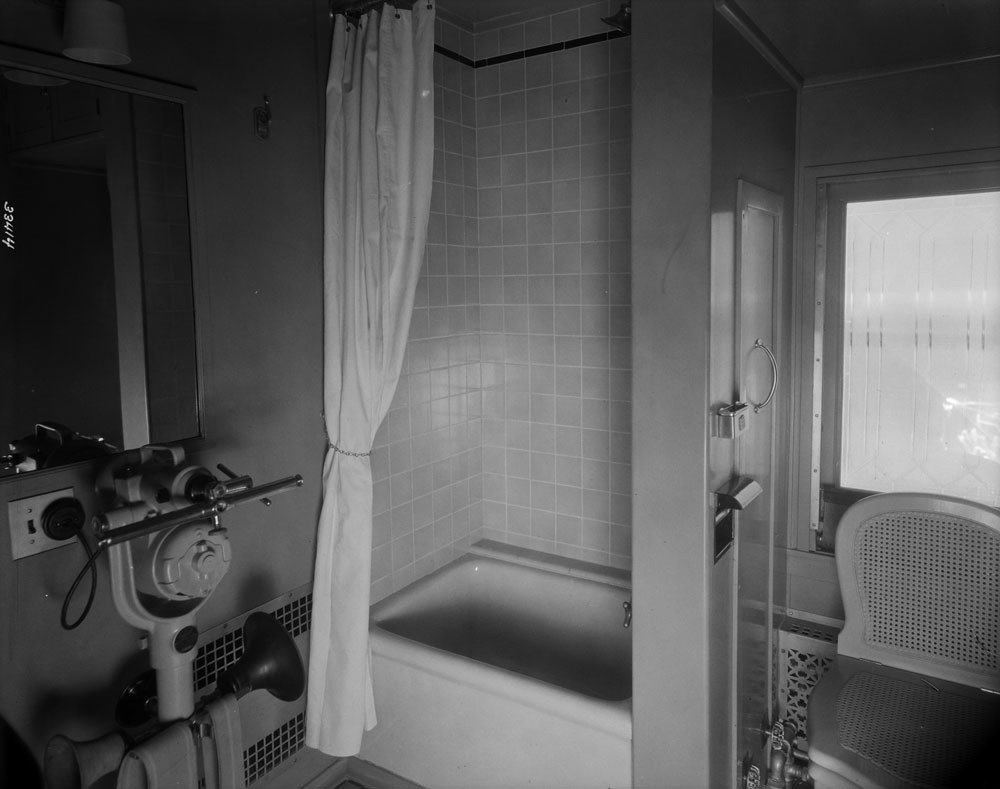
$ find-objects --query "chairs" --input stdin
[808,488,1000,789]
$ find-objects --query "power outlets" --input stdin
[7,485,80,562]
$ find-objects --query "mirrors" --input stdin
[0,46,207,483]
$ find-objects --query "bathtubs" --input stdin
[354,552,634,787]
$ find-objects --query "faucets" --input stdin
[620,596,634,628]
